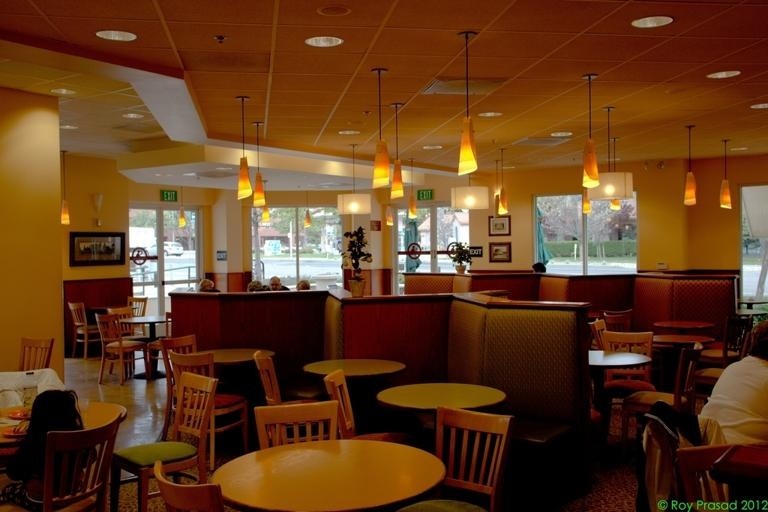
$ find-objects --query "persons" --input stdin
[699,320,768,445]
[199,276,310,292]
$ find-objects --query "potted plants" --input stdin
[450,241,473,273]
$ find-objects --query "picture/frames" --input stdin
[69,231,126,267]
[489,240,512,263]
[488,213,512,236]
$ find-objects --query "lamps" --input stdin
[59,149,75,225]
[92,193,104,226]
[216,28,733,227]
[179,176,187,228]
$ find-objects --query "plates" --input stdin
[2,423,35,437]
[2,406,40,419]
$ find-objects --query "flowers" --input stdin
[340,225,372,280]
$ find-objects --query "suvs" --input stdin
[143,241,183,256]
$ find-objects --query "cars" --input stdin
[259,238,321,253]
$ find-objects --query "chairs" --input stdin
[0,272,767,512]
